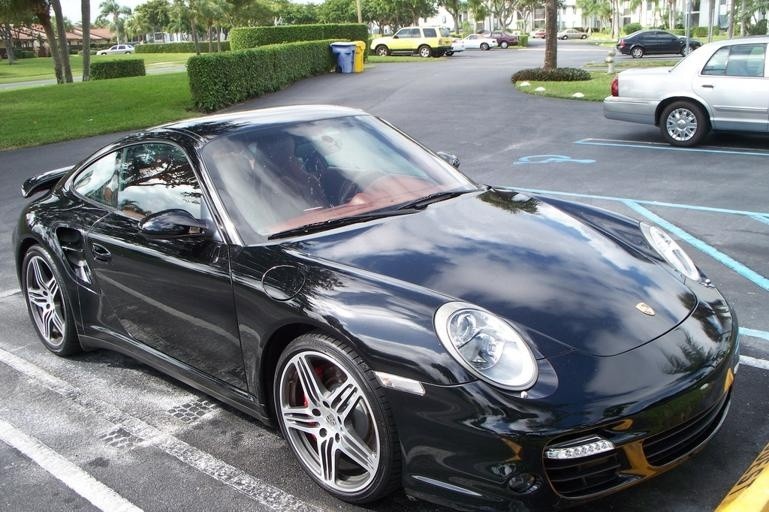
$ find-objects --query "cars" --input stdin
[534,29,546,38]
[464,34,497,50]
[602,35,769,147]
[484,32,519,48]
[557,28,587,39]
[616,29,701,59]
[95,44,135,55]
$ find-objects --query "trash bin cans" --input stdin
[330,42,356,73]
[353,41,366,72]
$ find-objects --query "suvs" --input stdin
[371,26,463,59]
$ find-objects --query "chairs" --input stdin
[253,132,331,224]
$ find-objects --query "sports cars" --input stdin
[13,104,739,509]
